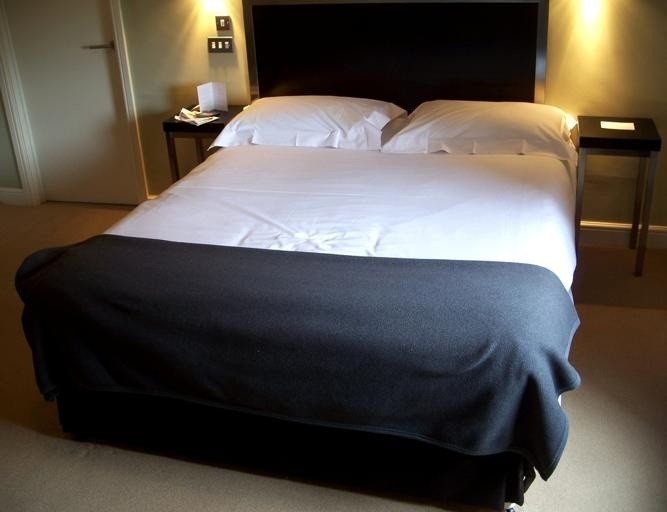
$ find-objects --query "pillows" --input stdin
[206,95,407,153]
[381,95,576,161]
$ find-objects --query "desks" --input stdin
[162,103,244,184]
[574,117,662,279]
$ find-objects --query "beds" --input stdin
[19,1,577,511]
